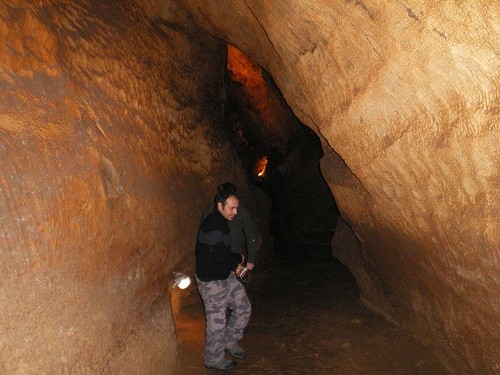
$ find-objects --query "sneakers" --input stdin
[205,358,236,370]
[226,342,247,359]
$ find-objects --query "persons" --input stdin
[195,184,254,372]
